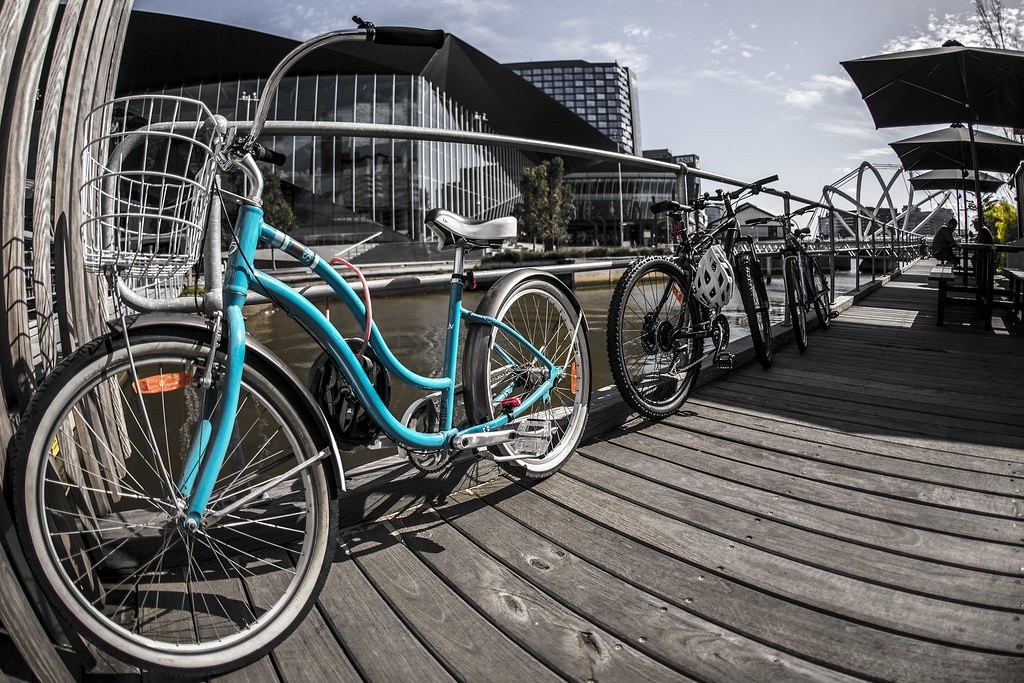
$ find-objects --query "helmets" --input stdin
[309,338,392,453]
[691,245,735,309]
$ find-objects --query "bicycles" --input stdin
[605,172,779,419]
[745,203,839,352]
[8,12,593,679]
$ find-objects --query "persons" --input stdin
[970,218,994,270]
[932,217,961,266]
[920,240,931,260]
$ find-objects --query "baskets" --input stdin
[80,95,223,276]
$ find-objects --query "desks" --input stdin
[955,243,1024,331]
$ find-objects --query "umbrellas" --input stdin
[888,122,1024,244]
[909,170,1005,244]
[839,39,1024,244]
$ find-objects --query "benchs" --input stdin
[928,273,955,326]
[937,257,947,265]
[944,266,953,273]
[1002,268,1020,301]
[1009,270,1024,327]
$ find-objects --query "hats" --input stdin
[971,217,978,222]
